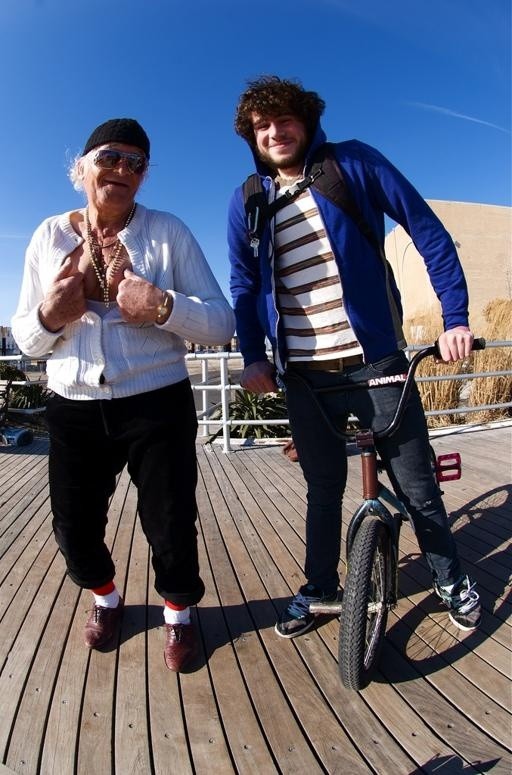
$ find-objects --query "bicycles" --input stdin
[228,332,489,690]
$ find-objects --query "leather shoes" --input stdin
[163,615,198,673]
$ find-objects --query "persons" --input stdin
[9,117,236,673]
[227,76,481,640]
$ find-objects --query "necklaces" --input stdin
[82,200,137,310]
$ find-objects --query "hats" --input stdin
[80,117,151,159]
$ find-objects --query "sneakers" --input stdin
[274,582,342,640]
[432,560,485,632]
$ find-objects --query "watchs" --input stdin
[157,289,171,326]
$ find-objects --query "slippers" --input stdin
[82,594,123,651]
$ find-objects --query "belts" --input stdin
[296,354,373,371]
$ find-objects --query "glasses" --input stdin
[85,148,148,176]
